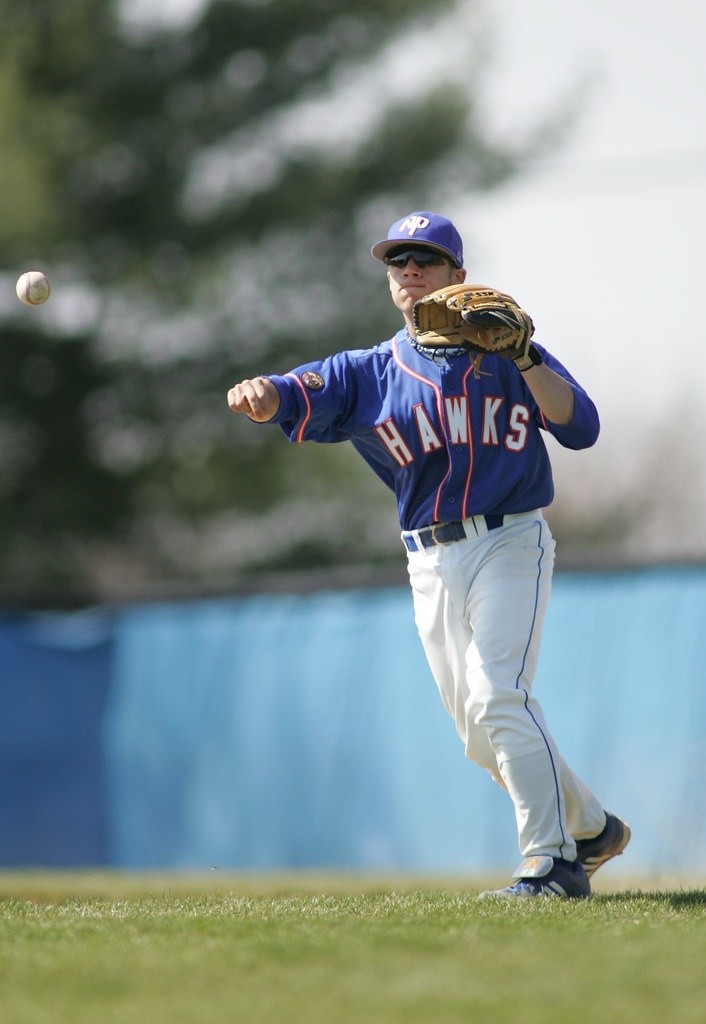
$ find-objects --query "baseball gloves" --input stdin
[409,283,533,376]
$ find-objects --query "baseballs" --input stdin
[16,269,49,308]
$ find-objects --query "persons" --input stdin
[228,213,631,901]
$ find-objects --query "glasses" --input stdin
[383,245,457,269]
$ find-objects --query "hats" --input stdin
[371,212,463,268]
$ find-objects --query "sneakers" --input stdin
[478,860,594,899]
[575,809,631,880]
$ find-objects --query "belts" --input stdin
[402,514,504,552]
